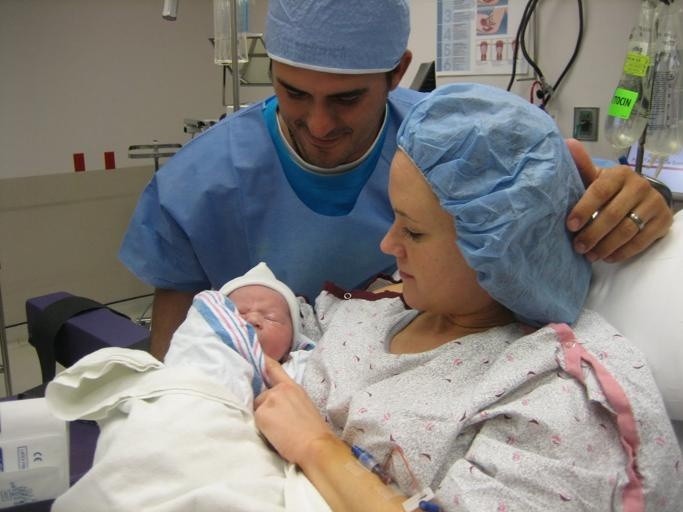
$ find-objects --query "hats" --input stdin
[218,260,301,351]
[264,0,410,74]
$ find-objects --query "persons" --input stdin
[50,83,682,511]
[117,0,674,364]
[216,262,299,364]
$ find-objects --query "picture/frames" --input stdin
[574,107,599,142]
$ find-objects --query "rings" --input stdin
[628,212,645,231]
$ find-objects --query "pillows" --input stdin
[584,208,683,421]
